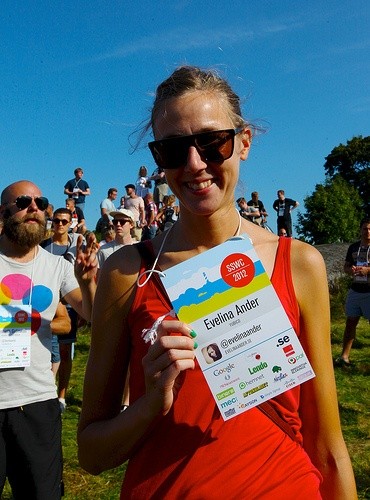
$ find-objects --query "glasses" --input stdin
[280,233,286,235]
[15,196,49,211]
[148,126,242,169]
[112,219,132,225]
[52,218,70,226]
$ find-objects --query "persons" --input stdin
[0,181,98,500]
[273,190,299,239]
[96,166,180,410]
[236,192,268,228]
[38,208,86,412]
[76,67,358,500]
[332,217,370,369]
[45,168,90,236]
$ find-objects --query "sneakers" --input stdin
[58,398,66,414]
[334,357,351,368]
[121,405,128,413]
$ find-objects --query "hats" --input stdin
[109,209,135,221]
[144,194,152,198]
